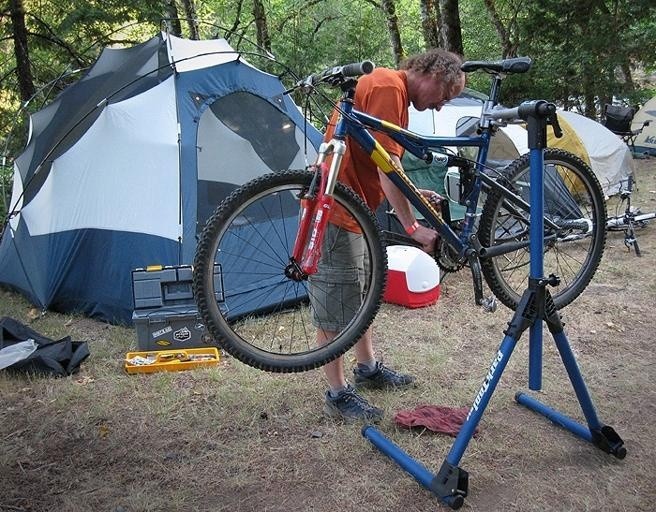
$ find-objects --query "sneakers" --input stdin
[324,384,383,421]
[354,361,412,388]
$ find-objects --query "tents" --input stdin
[1,28,325,325]
[373,87,655,242]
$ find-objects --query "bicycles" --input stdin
[552,175,656,258]
[192,56,609,374]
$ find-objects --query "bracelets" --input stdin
[406,222,419,235]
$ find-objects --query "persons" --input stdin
[301,48,465,423]
